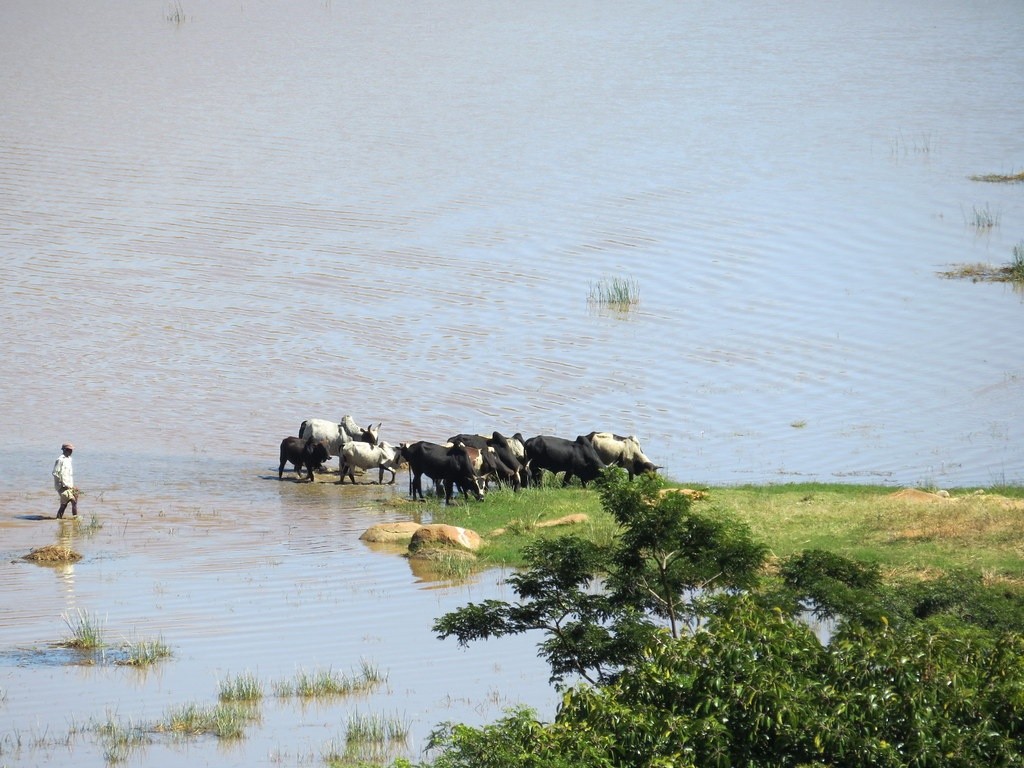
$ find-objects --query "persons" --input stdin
[52,444,78,518]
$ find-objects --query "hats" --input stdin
[62,443,74,449]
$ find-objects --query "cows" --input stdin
[279,415,665,506]
